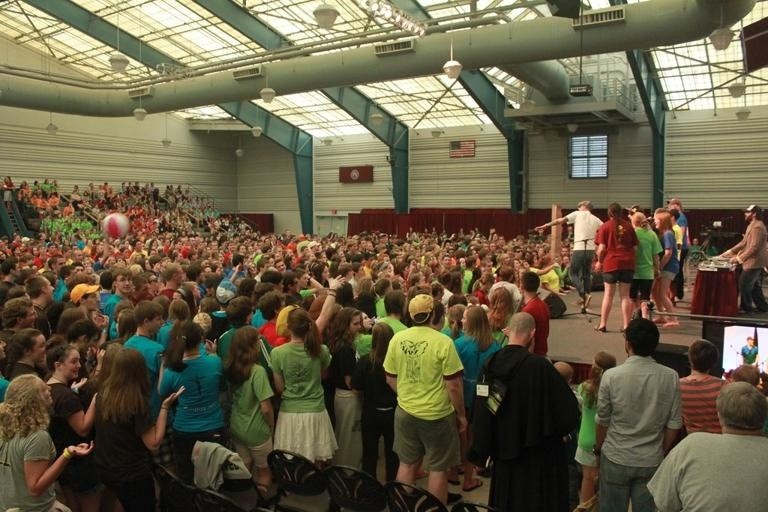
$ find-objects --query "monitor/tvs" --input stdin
[723,326,768,388]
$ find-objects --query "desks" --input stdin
[691,261,740,319]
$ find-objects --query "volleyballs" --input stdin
[103,212,129,238]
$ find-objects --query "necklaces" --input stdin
[51,375,65,384]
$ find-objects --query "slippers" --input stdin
[461,479,483,492]
[446,480,459,485]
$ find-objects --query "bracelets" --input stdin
[102,329,108,334]
[62,448,71,459]
[96,369,101,373]
[161,407,169,410]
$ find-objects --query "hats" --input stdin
[626,203,643,216]
[254,254,264,266]
[21,237,31,243]
[409,293,434,323]
[216,277,237,306]
[70,284,103,305]
[740,204,762,214]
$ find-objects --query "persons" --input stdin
[259,292,287,347]
[669,199,689,301]
[0,297,35,365]
[467,311,580,512]
[448,294,466,305]
[534,200,606,314]
[574,350,616,512]
[449,306,501,492]
[129,276,154,306]
[630,212,660,319]
[701,239,718,256]
[208,280,236,343]
[0,258,18,304]
[158,264,184,302]
[153,295,170,321]
[40,271,57,286]
[172,288,185,300]
[486,288,515,346]
[741,337,759,367]
[155,321,223,475]
[70,283,101,320]
[57,308,109,347]
[238,277,255,296]
[63,274,96,309]
[441,304,464,339]
[67,318,104,385]
[645,380,768,512]
[193,313,212,352]
[3,284,30,303]
[501,273,550,357]
[198,297,219,314]
[592,319,682,512]
[330,307,361,475]
[351,322,394,482]
[110,298,134,340]
[187,265,204,305]
[354,278,376,317]
[270,308,338,470]
[371,278,408,321]
[688,238,701,257]
[273,275,345,351]
[123,300,176,473]
[432,300,445,331]
[48,256,68,304]
[104,268,132,324]
[283,226,576,296]
[553,361,583,409]
[0,176,285,272]
[668,209,683,306]
[0,373,96,512]
[355,310,373,356]
[46,336,99,512]
[155,299,190,348]
[377,291,426,479]
[0,339,10,402]
[217,296,272,383]
[252,283,270,327]
[97,272,113,313]
[182,281,201,307]
[260,270,285,292]
[92,348,186,511]
[222,325,276,498]
[719,204,768,314]
[651,211,679,327]
[731,365,760,386]
[382,293,469,508]
[99,308,136,352]
[201,272,223,297]
[678,339,725,434]
[57,266,73,303]
[26,275,55,338]
[592,202,639,333]
[8,329,45,381]
[137,271,158,291]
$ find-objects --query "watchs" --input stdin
[592,444,599,455]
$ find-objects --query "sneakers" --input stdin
[412,464,429,477]
[573,297,587,305]
[663,321,680,328]
[652,316,666,323]
[581,293,593,308]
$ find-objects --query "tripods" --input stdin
[561,238,601,323]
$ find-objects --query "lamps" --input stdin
[133,1,147,121]
[443,1,463,79]
[709,0,734,50]
[312,0,340,30]
[431,106,443,138]
[43,0,60,135]
[567,121,579,133]
[236,134,245,158]
[728,70,748,98]
[259,1,276,101]
[324,125,333,146]
[108,0,129,71]
[736,96,751,121]
[251,98,263,137]
[370,97,383,126]
[162,110,171,147]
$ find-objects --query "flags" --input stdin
[450,141,475,157]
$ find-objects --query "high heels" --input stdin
[620,325,629,333]
[594,323,606,332]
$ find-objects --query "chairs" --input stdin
[193,487,247,512]
[323,463,388,510]
[152,463,189,509]
[450,500,495,512]
[266,450,338,511]
[195,440,281,509]
[384,480,449,512]
